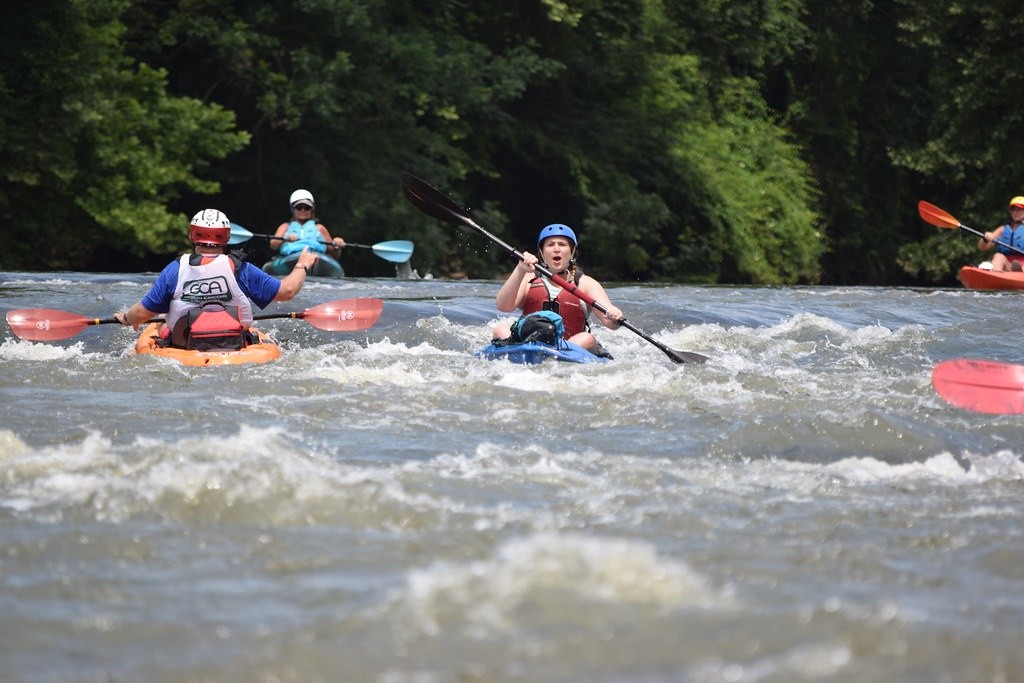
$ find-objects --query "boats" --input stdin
[477,311,606,370]
[957,253,1024,291]
[137,317,281,365]
[260,251,346,278]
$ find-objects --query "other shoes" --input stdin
[1011,260,1023,272]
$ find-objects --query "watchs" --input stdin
[294,263,309,276]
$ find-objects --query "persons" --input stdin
[493,222,624,354]
[978,195,1024,272]
[112,209,320,341]
[261,189,346,278]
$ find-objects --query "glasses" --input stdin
[295,204,311,210]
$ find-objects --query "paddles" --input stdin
[6,297,384,341]
[400,172,710,364]
[933,358,1024,415]
[918,199,1024,256]
[225,222,414,263]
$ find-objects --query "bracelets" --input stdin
[123,312,127,321]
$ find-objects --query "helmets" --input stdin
[1010,196,1024,205]
[290,189,314,204]
[190,208,231,247]
[538,224,578,249]
